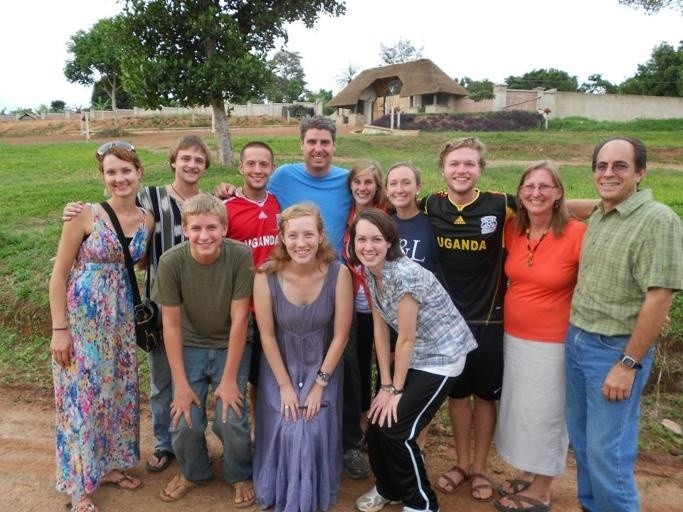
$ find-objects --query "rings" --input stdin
[285,404,290,409]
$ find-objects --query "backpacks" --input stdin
[101,201,161,352]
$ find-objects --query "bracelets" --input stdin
[379,384,393,390]
[51,327,67,331]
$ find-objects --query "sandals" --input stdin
[146,447,174,473]
[499,478,534,496]
[72,496,96,512]
[434,465,469,496]
[231,479,257,507]
[493,486,554,512]
[161,475,199,503]
[471,472,495,505]
[101,471,144,492]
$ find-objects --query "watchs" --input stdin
[389,385,404,395]
[318,370,330,382]
[616,350,643,371]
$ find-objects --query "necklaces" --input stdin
[526,216,553,266]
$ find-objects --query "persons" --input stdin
[48,142,152,507]
[220,143,281,419]
[254,203,353,512]
[347,207,479,512]
[566,137,682,512]
[496,164,670,509]
[419,137,602,501]
[61,136,218,472]
[341,161,388,445]
[150,194,255,507]
[214,118,375,480]
[383,164,435,272]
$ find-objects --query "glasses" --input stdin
[520,184,557,192]
[96,140,136,156]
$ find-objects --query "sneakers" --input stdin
[356,485,402,511]
[341,446,373,480]
